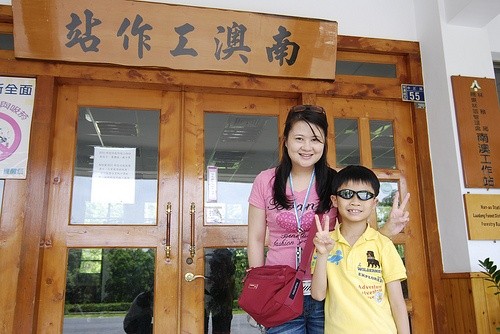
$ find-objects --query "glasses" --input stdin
[291,104,325,113]
[336,189,374,201]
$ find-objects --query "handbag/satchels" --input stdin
[238,265,304,329]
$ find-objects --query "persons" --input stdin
[122,289,154,334]
[204,246,240,334]
[309,163,411,334]
[245,102,409,334]
